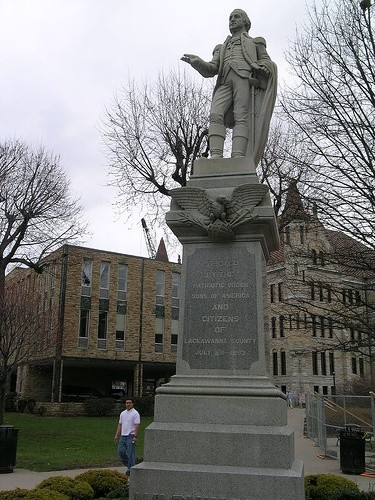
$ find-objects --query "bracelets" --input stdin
[134,436,138,438]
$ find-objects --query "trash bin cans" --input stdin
[0,425,19,474]
[340,424,366,475]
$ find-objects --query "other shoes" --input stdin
[126,471,130,476]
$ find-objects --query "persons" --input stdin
[180,9,271,158]
[287,391,293,409]
[114,399,140,475]
